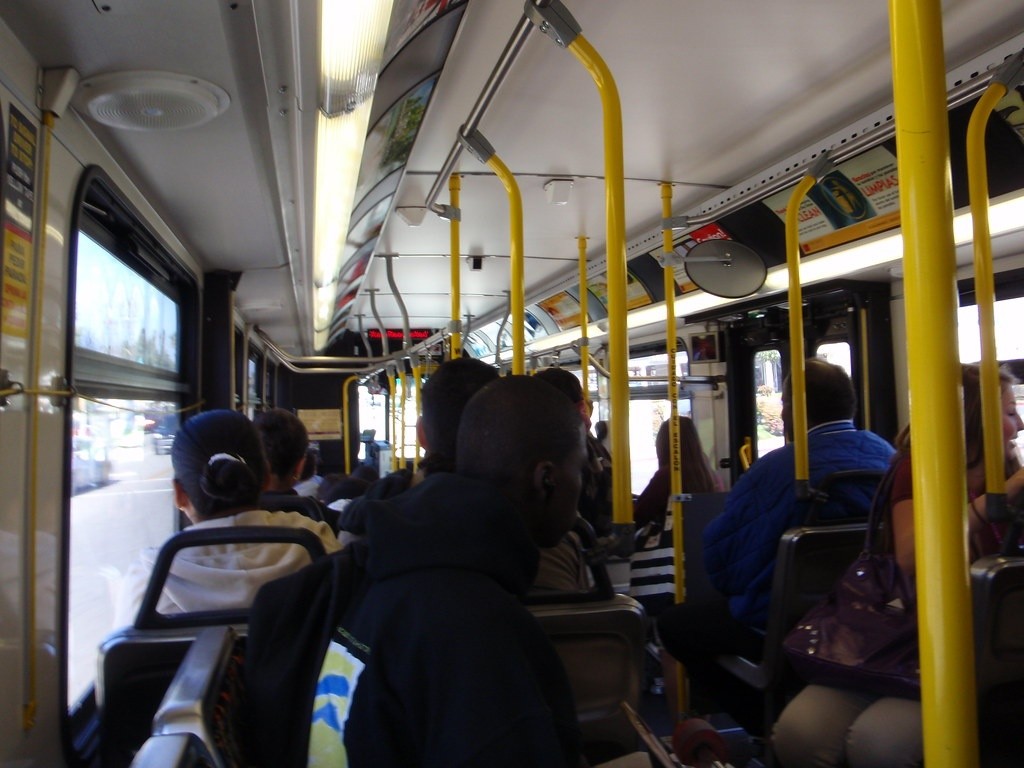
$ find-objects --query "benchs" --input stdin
[620,491,1023,768]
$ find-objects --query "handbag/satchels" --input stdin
[781,551,920,697]
[628,519,676,597]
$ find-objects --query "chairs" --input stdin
[95,526,656,767]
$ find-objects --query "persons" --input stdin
[156,356,1024,768]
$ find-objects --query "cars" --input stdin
[71,434,114,491]
[155,439,176,455]
